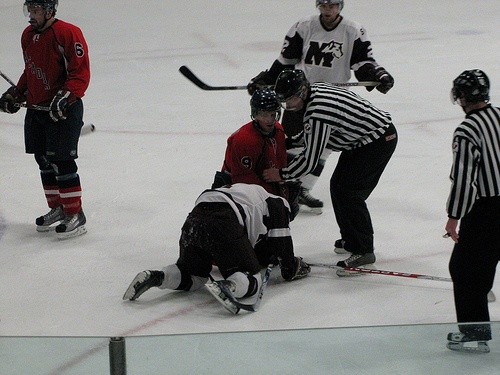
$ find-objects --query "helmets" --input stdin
[452,68,490,106]
[23,0,58,11]
[314,0,344,7]
[273,68,308,101]
[248,86,282,114]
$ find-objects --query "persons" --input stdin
[245,0,394,215]
[213,89,299,222]
[444,69,500,353]
[123,182,310,317]
[0,0,90,238]
[261,69,398,267]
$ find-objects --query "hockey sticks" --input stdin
[306,261,497,304]
[217,263,275,313]
[178,65,381,91]
[11,101,51,112]
[0,70,97,137]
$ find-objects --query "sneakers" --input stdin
[296,186,324,215]
[122,266,162,304]
[336,252,376,278]
[333,237,354,254]
[54,208,87,239]
[443,322,492,354]
[204,279,242,316]
[35,206,67,232]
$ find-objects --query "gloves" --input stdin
[374,67,394,94]
[298,257,311,279]
[0,86,25,114]
[47,90,72,122]
[247,70,272,95]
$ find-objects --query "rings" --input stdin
[443,233,451,237]
